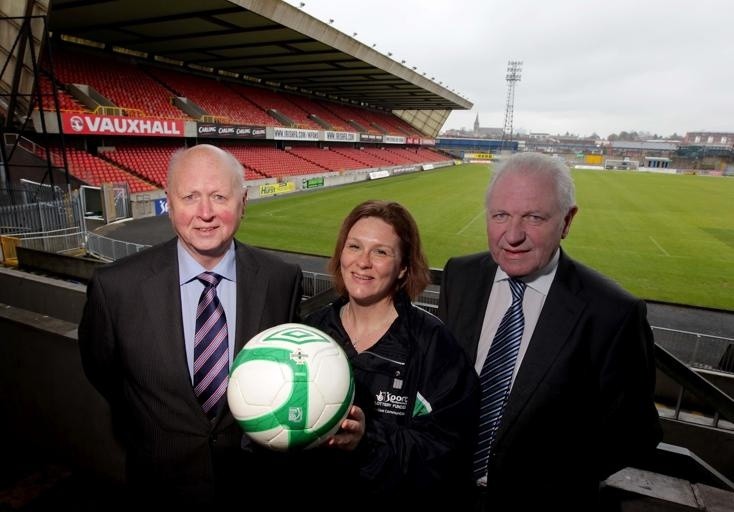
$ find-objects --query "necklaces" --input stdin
[342,310,389,346]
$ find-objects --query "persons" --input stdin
[433,139,662,512]
[74,142,308,510]
[239,198,457,510]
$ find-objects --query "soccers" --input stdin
[227,323,355,452]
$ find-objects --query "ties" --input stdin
[471,279,525,480]
[192,271,230,421]
